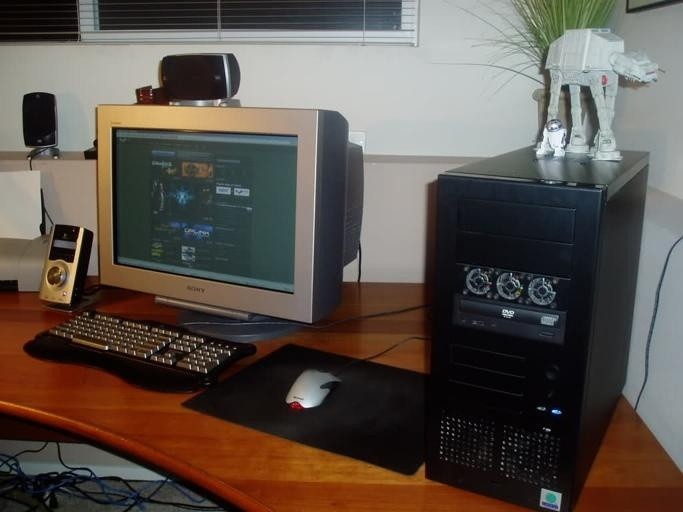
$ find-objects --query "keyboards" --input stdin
[22,308,257,388]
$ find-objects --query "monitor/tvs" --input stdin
[97,106,362,344]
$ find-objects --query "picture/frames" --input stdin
[625,1,683,15]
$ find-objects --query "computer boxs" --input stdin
[424,140,649,512]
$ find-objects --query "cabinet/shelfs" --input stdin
[0,278,683,511]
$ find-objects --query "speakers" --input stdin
[22,91,59,147]
[159,54,240,99]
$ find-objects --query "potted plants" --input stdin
[446,1,614,144]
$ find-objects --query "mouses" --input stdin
[285,368,341,410]
